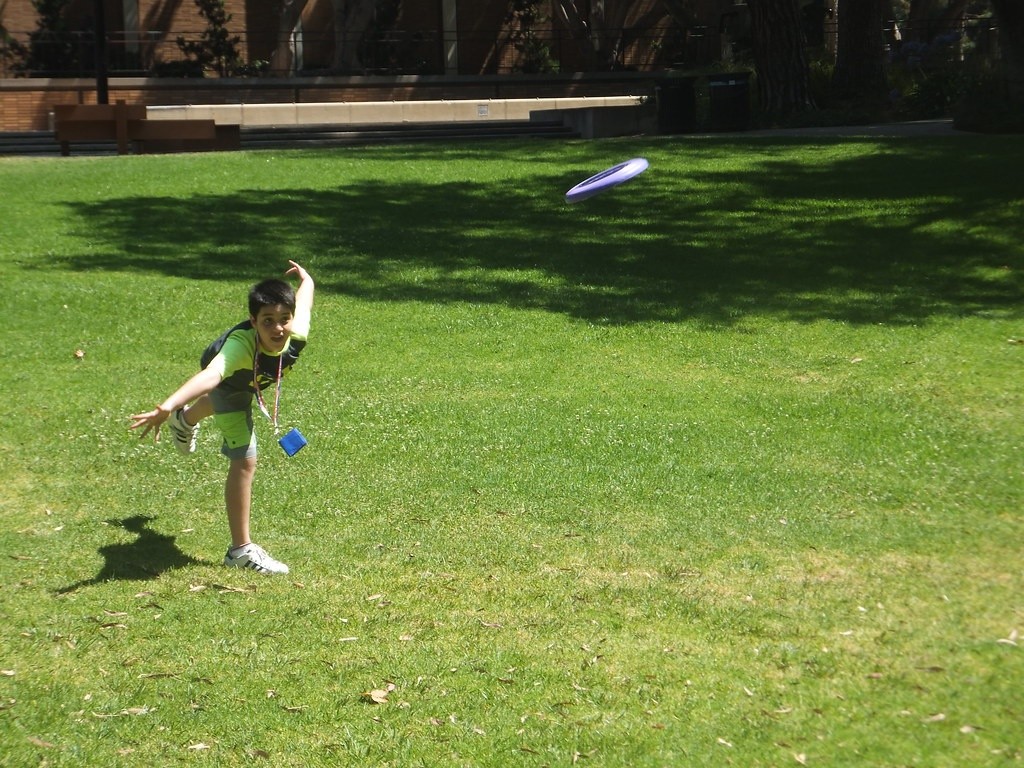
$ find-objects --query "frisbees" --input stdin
[564,156,649,204]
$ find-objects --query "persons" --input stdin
[130,260,315,575]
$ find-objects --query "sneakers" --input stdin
[166,404,200,455]
[222,542,289,575]
